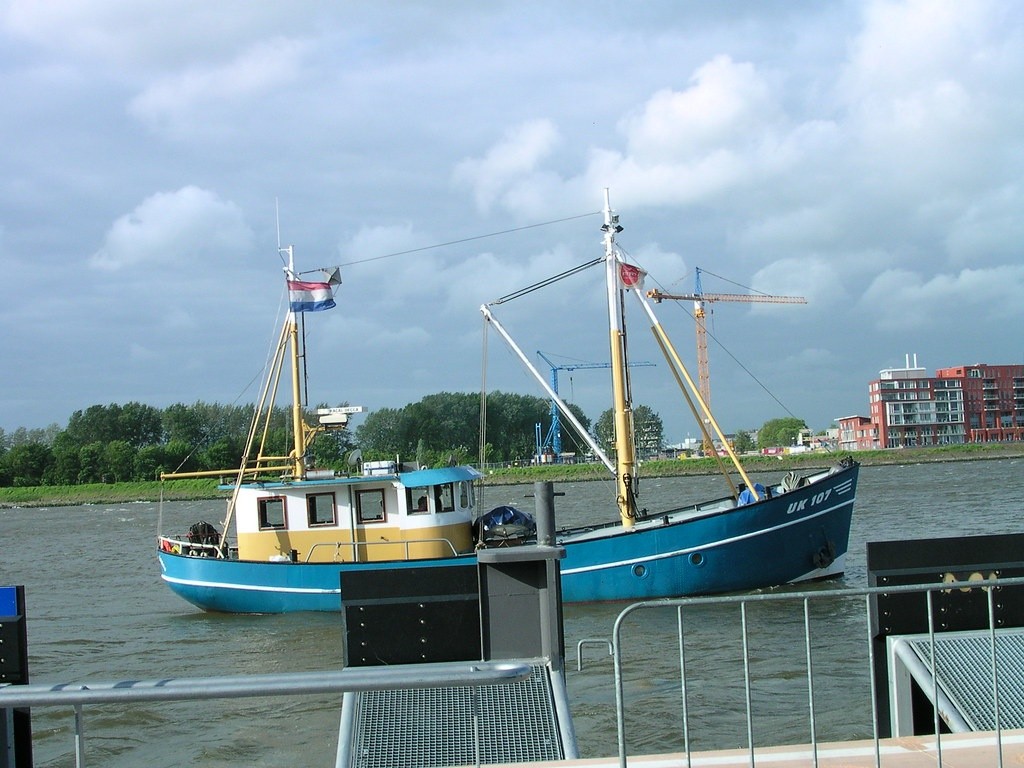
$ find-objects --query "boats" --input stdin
[153,245,489,614]
[476,188,861,600]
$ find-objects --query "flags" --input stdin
[613,257,649,290]
[286,279,336,313]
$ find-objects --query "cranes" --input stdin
[644,265,809,455]
[535,350,658,461]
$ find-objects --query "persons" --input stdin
[418,496,427,510]
[433,483,444,512]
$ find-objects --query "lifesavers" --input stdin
[171,545,180,554]
[163,541,171,552]
